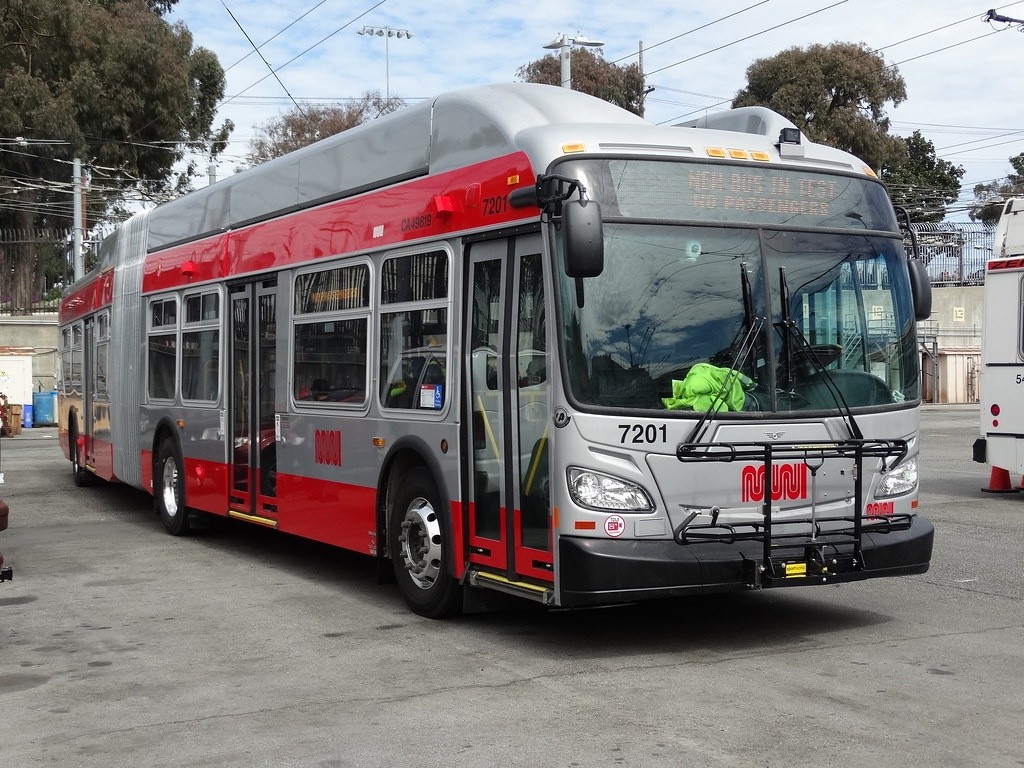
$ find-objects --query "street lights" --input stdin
[356,26,415,114]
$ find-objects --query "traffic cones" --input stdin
[1014,475,1024,491]
[978,465,1020,493]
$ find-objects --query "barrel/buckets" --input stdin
[33,392,58,425]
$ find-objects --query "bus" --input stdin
[971,198,1024,477]
[56,80,938,622]
